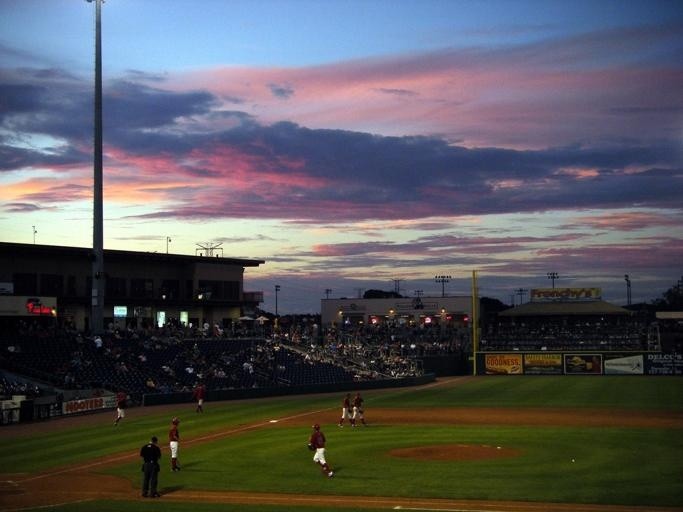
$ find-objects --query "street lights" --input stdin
[167,236,171,254]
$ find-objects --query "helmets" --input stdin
[313,424,320,429]
[151,436,158,441]
[346,392,359,396]
[172,417,180,425]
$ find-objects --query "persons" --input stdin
[338,393,357,428]
[112,391,132,427]
[168,417,182,472]
[309,423,336,480]
[351,393,368,427]
[191,382,206,412]
[139,435,162,498]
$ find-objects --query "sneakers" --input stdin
[143,493,161,497]
[337,424,368,428]
[171,467,180,471]
